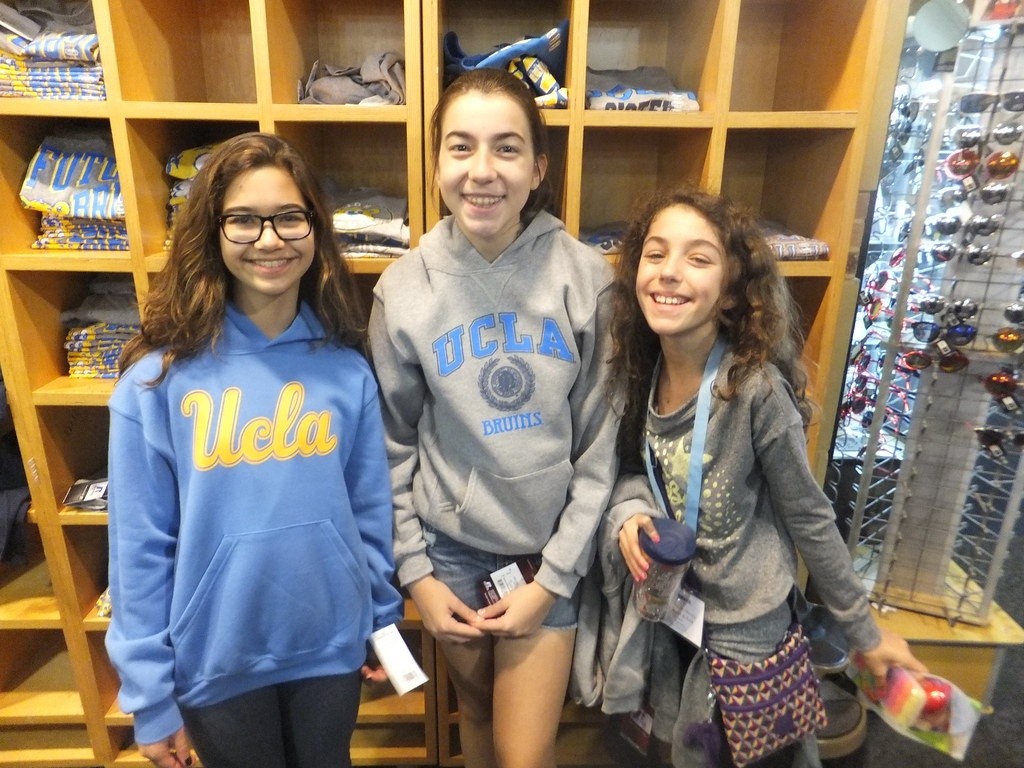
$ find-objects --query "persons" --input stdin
[607,189,929,768]
[368,68,628,768]
[104,133,407,768]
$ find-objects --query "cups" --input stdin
[636,518,697,622]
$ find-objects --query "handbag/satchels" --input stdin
[681,623,828,768]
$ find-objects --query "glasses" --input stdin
[822,49,1024,489]
[215,210,315,245]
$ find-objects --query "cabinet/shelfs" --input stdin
[0,0,1024,768]
